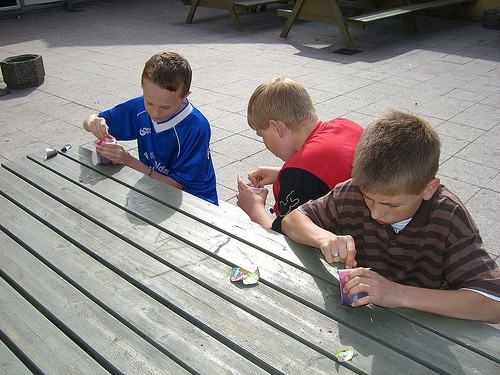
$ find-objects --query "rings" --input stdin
[331,254,339,258]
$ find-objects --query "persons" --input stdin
[84,52,218,206]
[281,110,500,324]
[237,77,364,234]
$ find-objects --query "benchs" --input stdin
[232,0,278,13]
[348,0,465,30]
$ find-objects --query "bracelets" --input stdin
[148,166,152,176]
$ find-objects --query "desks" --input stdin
[182,0,465,51]
[0,142,500,375]
